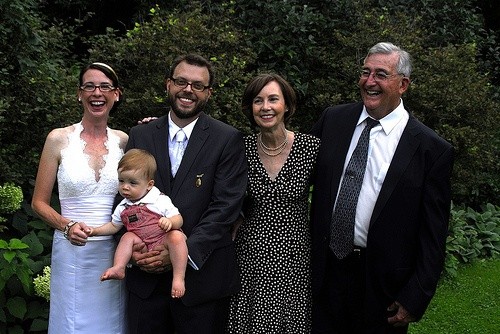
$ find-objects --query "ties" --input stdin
[328,117,380,261]
[171,131,187,178]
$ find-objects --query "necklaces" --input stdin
[257,128,289,156]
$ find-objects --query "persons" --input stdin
[231,71,321,334]
[81,149,189,298]
[32,59,159,333]
[124,52,246,333]
[309,41,447,331]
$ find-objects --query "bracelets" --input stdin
[63,220,78,239]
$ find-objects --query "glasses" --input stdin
[80,83,116,93]
[171,77,210,93]
[356,69,407,82]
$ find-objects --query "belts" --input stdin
[349,247,365,259]
